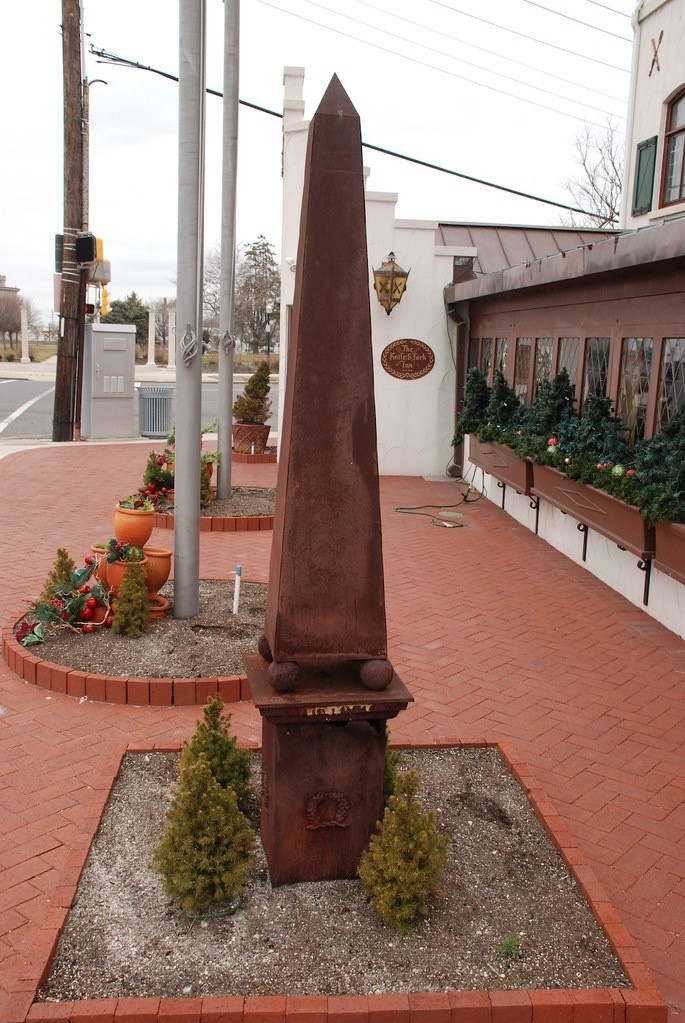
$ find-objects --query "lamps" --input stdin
[372,252,412,316]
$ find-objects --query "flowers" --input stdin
[96,537,144,563]
[450,365,685,529]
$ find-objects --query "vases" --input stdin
[106,555,149,597]
[467,432,534,496]
[653,519,685,586]
[146,594,168,619]
[141,547,172,596]
[530,460,656,558]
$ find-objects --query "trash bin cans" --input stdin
[135,381,176,440]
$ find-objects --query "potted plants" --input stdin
[232,360,273,454]
[114,419,223,550]
[92,542,110,588]
